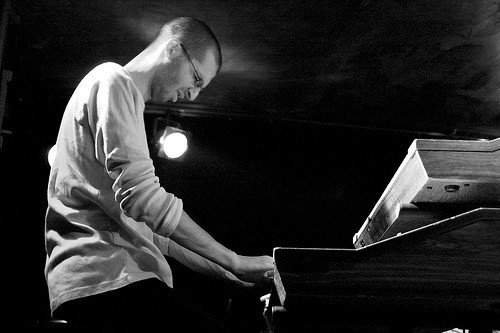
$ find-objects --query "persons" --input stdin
[44,15,275,333]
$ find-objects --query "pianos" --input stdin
[253,136,499,333]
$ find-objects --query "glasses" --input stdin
[180,43,207,94]
